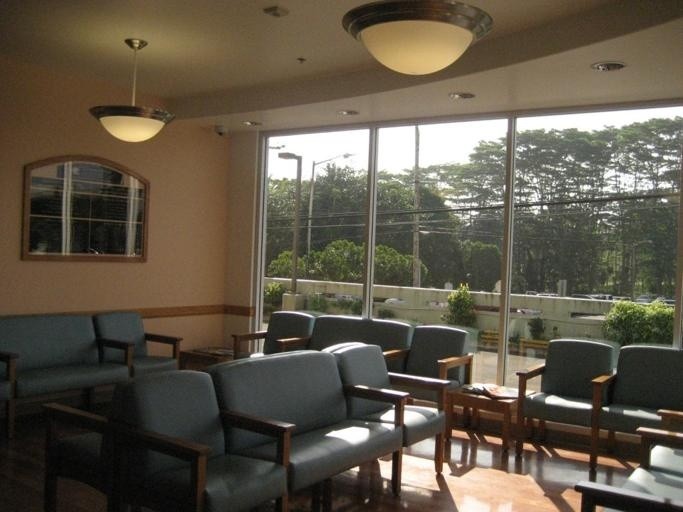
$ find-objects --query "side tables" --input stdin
[444,381,536,461]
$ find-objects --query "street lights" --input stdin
[308,150,362,283]
[277,151,305,293]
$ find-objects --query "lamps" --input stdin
[341,0,494,76]
[89,38,176,143]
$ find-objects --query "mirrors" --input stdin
[21,154,151,262]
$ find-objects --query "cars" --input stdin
[384,297,406,304]
[517,290,676,314]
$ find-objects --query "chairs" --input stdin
[0,310,474,512]
[574,344,683,512]
[514,338,616,483]
